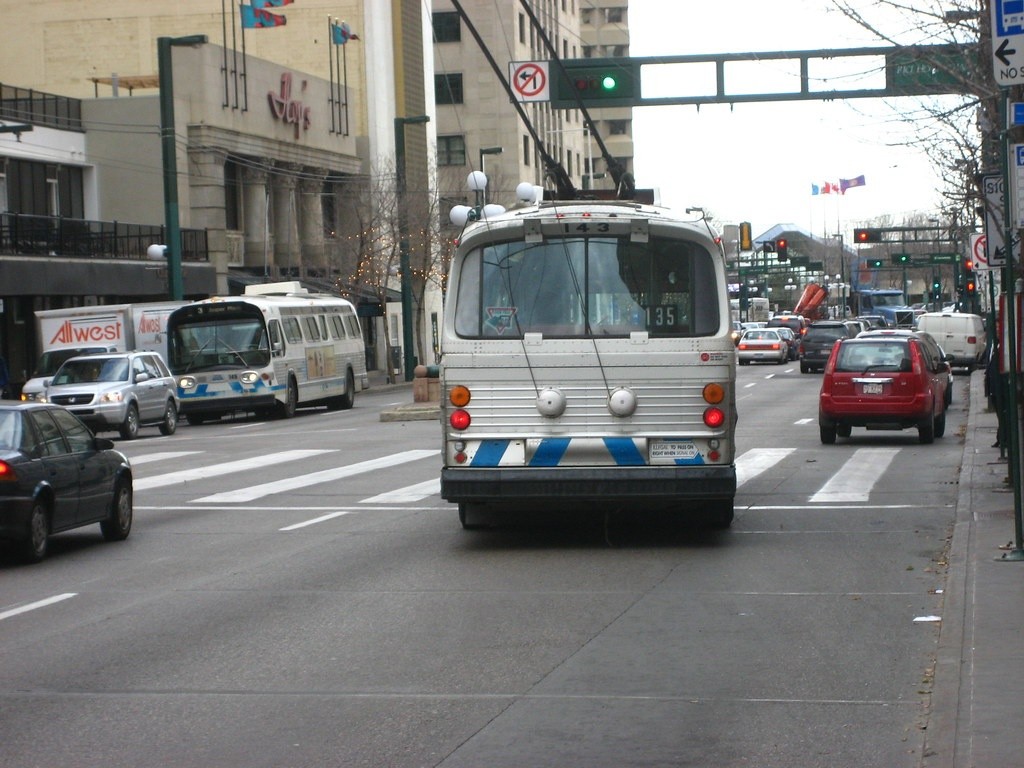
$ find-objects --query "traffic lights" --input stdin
[777,239,788,262]
[867,259,884,268]
[964,259,976,296]
[894,252,913,265]
[854,228,881,243]
[933,275,940,291]
[550,56,638,107]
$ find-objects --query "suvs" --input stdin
[818,335,949,446]
[845,326,954,406]
[800,322,851,373]
[42,350,181,440]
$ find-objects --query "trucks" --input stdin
[850,288,915,330]
[20,299,196,405]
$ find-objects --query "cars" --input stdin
[733,302,957,362]
[737,327,789,365]
[1,399,133,564]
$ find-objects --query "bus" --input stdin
[438,0,738,533]
[155,280,369,426]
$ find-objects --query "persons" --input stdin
[986,317,1013,447]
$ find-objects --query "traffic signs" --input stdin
[991,1,1024,87]
[983,174,1020,267]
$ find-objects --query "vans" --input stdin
[916,312,987,375]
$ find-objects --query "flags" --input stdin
[332,24,362,44]
[240,4,287,29]
[811,175,866,197]
[250,0,294,8]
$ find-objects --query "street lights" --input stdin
[480,146,505,209]
[835,274,841,321]
[927,218,942,311]
[155,35,209,301]
[784,278,797,311]
[832,234,845,319]
[393,116,431,382]
[824,274,830,320]
